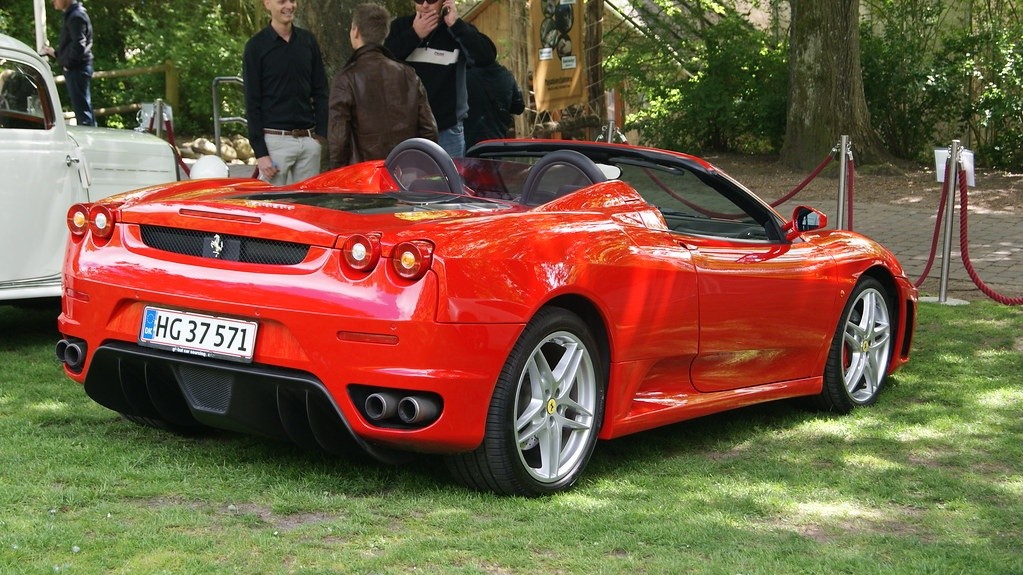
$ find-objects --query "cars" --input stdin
[0,33,230,316]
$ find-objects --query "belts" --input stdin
[263,128,315,137]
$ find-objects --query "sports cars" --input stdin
[57,136,919,497]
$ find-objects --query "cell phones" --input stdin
[441,6,448,17]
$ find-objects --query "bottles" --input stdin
[255,164,279,183]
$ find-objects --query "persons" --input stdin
[383,0,498,176]
[43,0,95,126]
[242,0,328,186]
[328,4,438,174]
[463,62,526,168]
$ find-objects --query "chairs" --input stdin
[384,137,464,194]
[521,149,606,205]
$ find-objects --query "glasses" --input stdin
[415,0,438,4]
